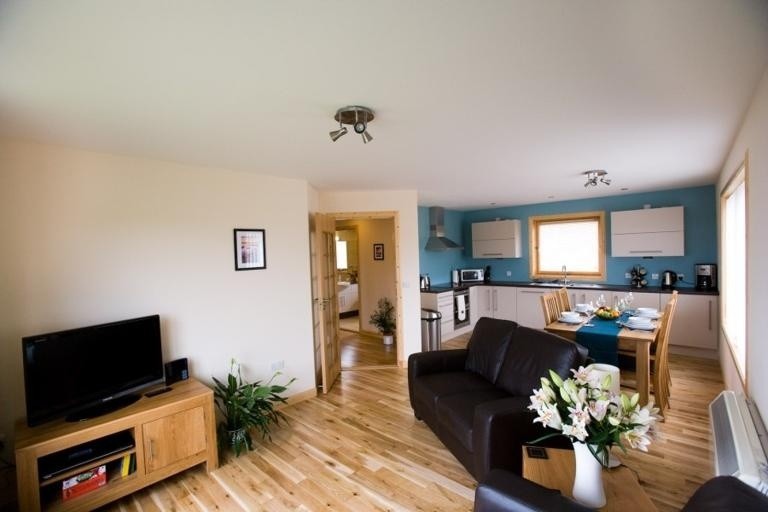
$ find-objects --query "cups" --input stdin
[595,293,606,308]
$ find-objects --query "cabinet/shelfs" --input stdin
[611,292,660,310]
[12,377,219,511]
[469,285,517,333]
[610,203,685,259]
[520,446,662,512]
[565,289,610,308]
[471,219,522,260]
[660,296,719,352]
[515,288,547,329]
[421,291,454,343]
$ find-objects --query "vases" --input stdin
[568,436,607,508]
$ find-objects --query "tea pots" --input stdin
[420,273,430,288]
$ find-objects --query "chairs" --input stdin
[536,292,560,323]
[617,294,678,423]
[555,286,571,310]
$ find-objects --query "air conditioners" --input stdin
[707,388,767,494]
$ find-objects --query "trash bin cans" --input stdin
[421,308,442,353]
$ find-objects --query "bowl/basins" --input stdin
[627,316,651,325]
[560,311,579,319]
[575,303,586,311]
[638,307,657,316]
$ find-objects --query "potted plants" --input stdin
[367,296,397,346]
[209,358,296,455]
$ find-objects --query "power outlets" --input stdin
[676,274,683,281]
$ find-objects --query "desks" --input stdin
[545,309,663,409]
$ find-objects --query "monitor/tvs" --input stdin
[22,314,166,427]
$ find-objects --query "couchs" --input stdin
[465,462,767,511]
[407,318,594,479]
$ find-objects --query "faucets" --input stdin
[562,265,567,283]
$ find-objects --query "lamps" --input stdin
[580,168,612,190]
[328,106,374,144]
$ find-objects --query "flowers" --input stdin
[525,370,666,473]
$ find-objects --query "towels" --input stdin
[455,295,465,320]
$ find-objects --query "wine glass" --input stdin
[614,296,626,324]
[624,290,635,315]
[582,300,595,327]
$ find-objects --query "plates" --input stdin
[557,317,581,324]
[645,313,662,319]
[624,322,657,330]
[575,307,586,314]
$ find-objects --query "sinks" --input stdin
[569,282,600,290]
[530,281,570,289]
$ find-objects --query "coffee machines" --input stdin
[696,264,717,289]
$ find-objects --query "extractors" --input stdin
[423,207,465,252]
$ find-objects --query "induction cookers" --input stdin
[432,283,471,290]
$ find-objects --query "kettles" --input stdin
[661,271,677,290]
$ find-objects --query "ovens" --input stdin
[453,288,470,330]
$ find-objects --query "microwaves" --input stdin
[459,269,484,282]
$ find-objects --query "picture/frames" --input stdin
[232,228,267,271]
[372,242,384,261]
